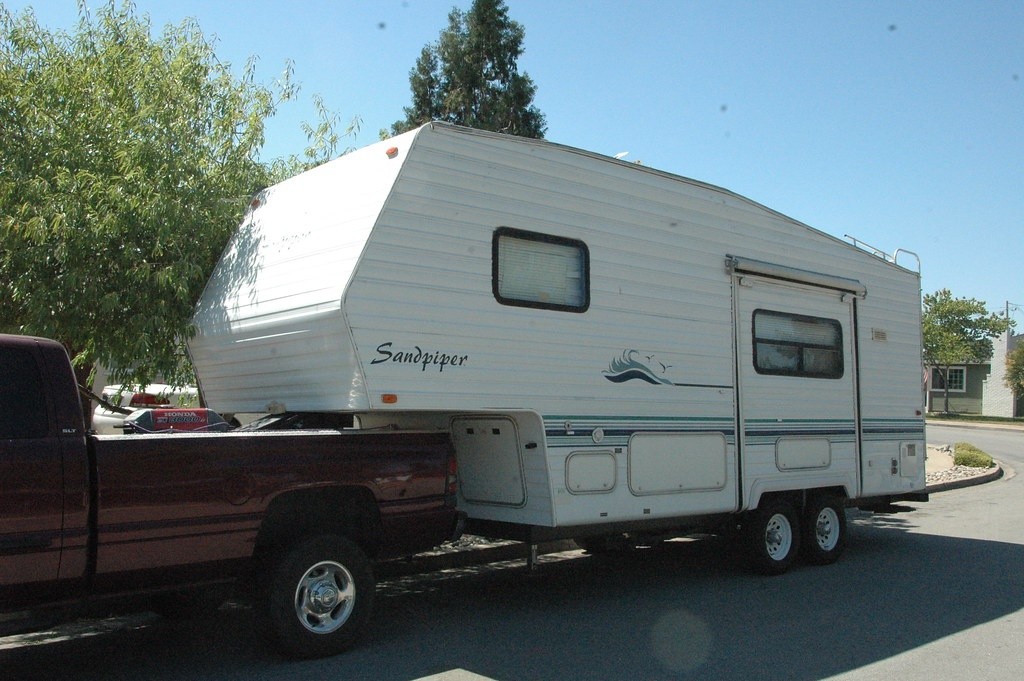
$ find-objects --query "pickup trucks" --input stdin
[0,329,467,660]
